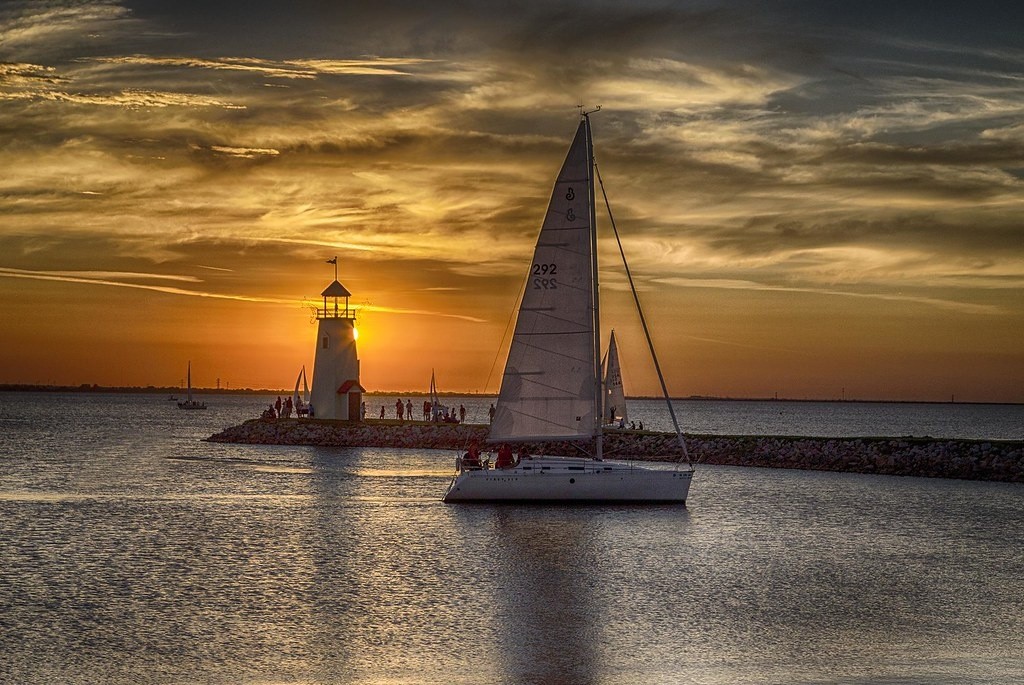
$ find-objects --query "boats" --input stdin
[163,395,178,401]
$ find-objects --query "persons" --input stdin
[380,406,385,419]
[182,400,204,406]
[495,444,515,469]
[463,444,482,471]
[488,404,495,425]
[609,405,616,426]
[618,418,625,429]
[263,396,293,418]
[459,404,466,423]
[308,403,314,418]
[636,421,643,430]
[515,447,533,467]
[295,396,303,418]
[628,421,635,430]
[396,399,460,423]
[360,401,366,422]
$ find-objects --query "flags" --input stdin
[327,258,336,264]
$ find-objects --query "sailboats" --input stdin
[293,365,311,406]
[177,360,208,409]
[430,368,449,410]
[600,329,629,429]
[441,104,704,504]
